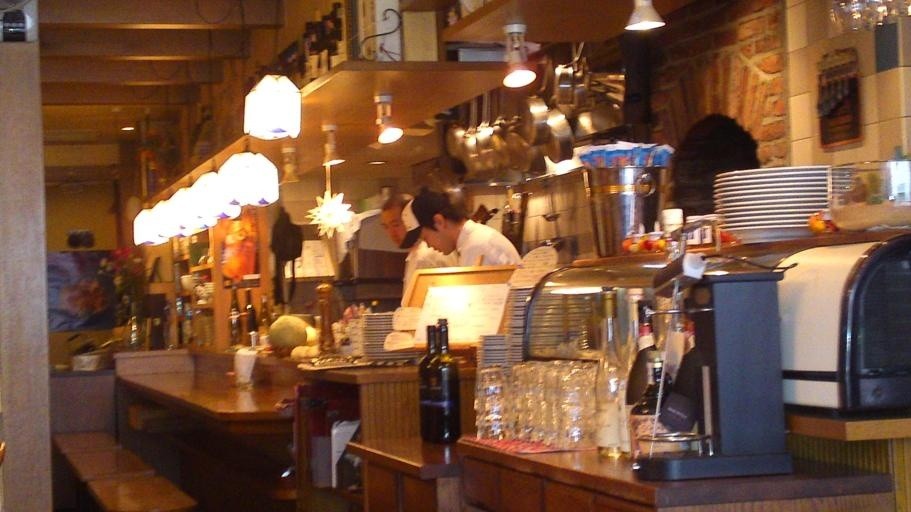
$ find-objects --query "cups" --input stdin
[472,362,595,451]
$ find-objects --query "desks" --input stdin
[115,372,297,435]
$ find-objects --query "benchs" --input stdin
[54,429,198,512]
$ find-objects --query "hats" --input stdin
[399,186,449,249]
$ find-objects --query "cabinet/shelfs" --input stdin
[141,209,276,351]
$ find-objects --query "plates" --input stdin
[710,159,881,243]
[357,309,421,361]
[472,282,595,372]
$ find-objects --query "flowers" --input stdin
[94,245,144,325]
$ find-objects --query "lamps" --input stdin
[131,0,403,248]
[624,0,667,31]
[501,24,537,88]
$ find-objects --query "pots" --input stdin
[442,40,597,185]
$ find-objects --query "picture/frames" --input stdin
[817,74,863,148]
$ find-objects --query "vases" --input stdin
[112,325,130,349]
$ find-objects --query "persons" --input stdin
[398,191,521,266]
[380,193,459,306]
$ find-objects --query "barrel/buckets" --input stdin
[578,164,667,257]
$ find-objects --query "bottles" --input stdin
[596,282,693,466]
[659,205,717,245]
[416,317,464,445]
[125,221,278,348]
